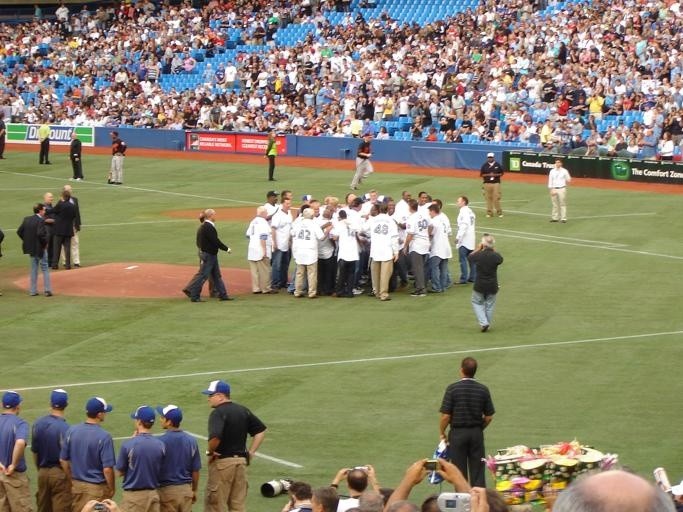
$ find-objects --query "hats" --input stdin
[267,191,280,197]
[377,195,388,204]
[156,404,182,422]
[201,380,231,396]
[51,389,68,408]
[3,391,24,408]
[85,396,112,413]
[361,193,371,200]
[487,152,495,158]
[131,405,155,423]
[302,194,312,201]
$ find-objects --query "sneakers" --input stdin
[253,279,473,301]
[481,324,490,332]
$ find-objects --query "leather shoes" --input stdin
[220,297,234,301]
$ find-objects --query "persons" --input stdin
[466,234,504,333]
[199,378,267,512]
[545,156,572,224]
[0,390,31,511]
[79,494,119,511]
[14,183,82,297]
[479,152,504,219]
[57,394,116,511]
[152,401,201,511]
[28,385,71,511]
[113,405,166,511]
[435,354,495,495]
[183,184,480,303]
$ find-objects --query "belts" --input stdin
[555,186,566,189]
[217,451,247,460]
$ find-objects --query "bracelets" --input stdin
[204,450,213,456]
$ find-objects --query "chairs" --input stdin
[160,20,272,92]
[498,109,682,162]
[274,0,478,49]
[4,44,149,108]
[361,113,498,146]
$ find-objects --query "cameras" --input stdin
[424,459,440,472]
[435,492,470,512]
[347,467,368,474]
[94,503,106,511]
